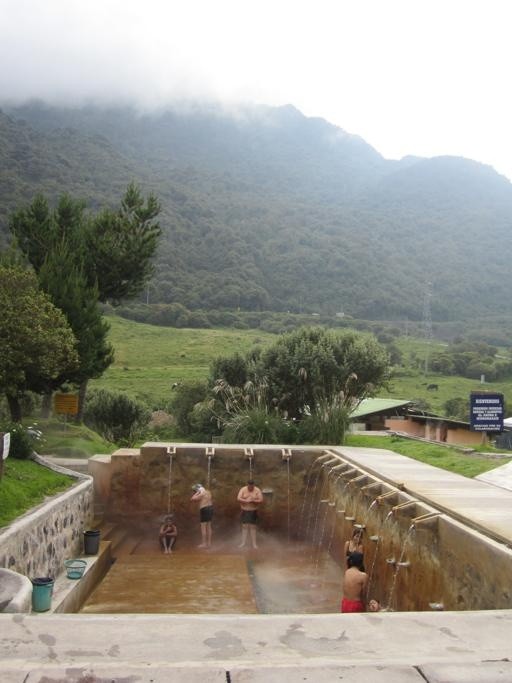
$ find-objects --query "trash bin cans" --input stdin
[83,530,100,554]
[32,577,54,612]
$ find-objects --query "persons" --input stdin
[189,482,214,548]
[368,598,389,611]
[340,551,371,612]
[158,515,179,554]
[236,478,264,550]
[343,528,365,572]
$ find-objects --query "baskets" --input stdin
[65,559,88,579]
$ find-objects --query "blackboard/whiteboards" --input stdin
[470,392,503,432]
